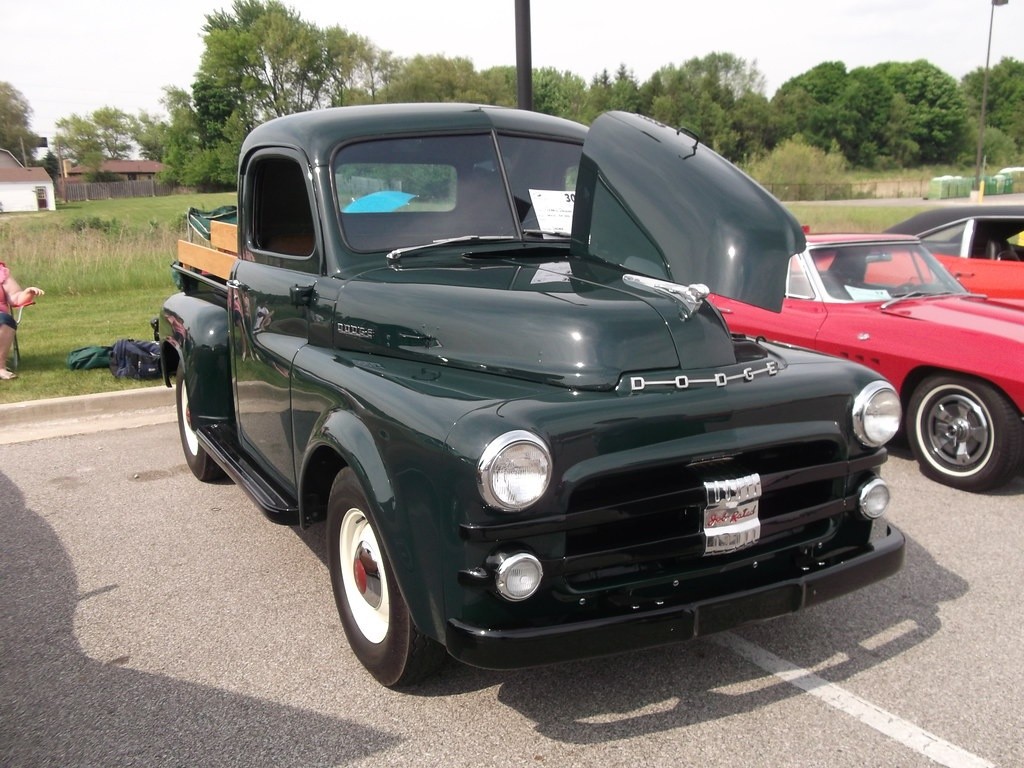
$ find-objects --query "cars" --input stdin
[704,234,1024,490]
[791,203,1024,300]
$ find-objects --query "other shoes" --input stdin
[0,370,18,381]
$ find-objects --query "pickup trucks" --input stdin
[151,103,907,689]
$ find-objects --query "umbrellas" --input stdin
[340,190,419,213]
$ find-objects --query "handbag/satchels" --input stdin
[67,345,112,370]
[109,339,161,380]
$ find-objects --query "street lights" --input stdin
[975,0,1009,190]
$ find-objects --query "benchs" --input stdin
[267,231,483,258]
[924,240,986,261]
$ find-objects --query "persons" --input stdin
[0,260,46,380]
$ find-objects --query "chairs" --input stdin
[987,238,1021,261]
